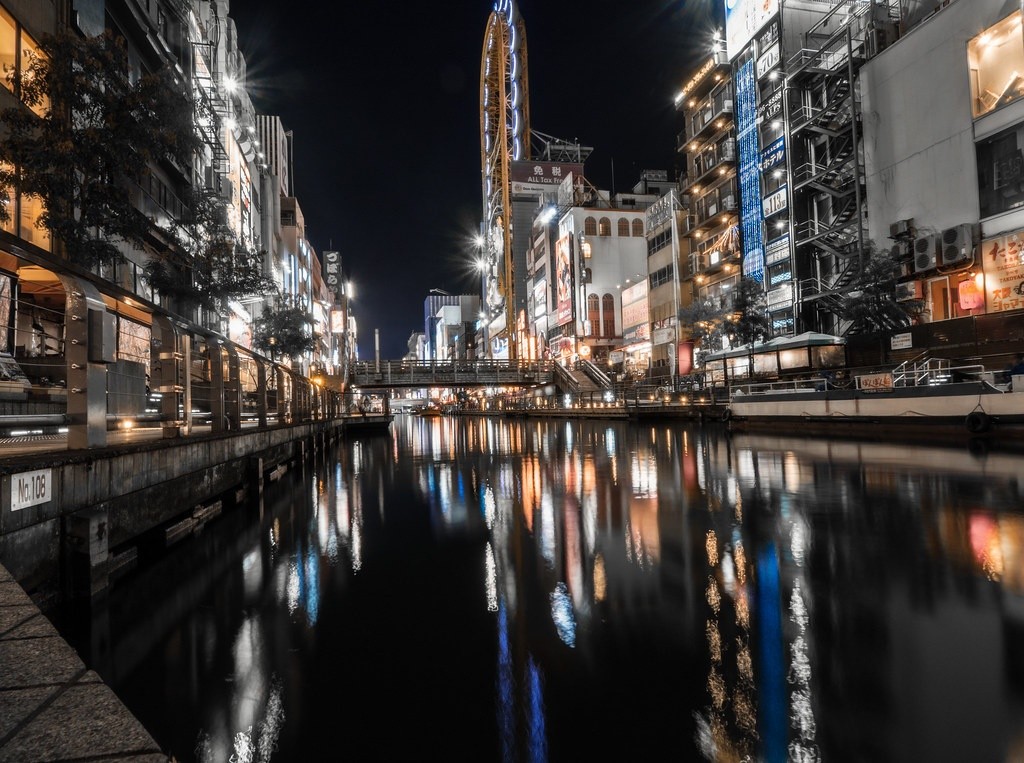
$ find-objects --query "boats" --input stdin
[421,405,440,416]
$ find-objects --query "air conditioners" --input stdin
[721,137,735,158]
[889,218,974,303]
[687,251,710,276]
[721,195,735,209]
